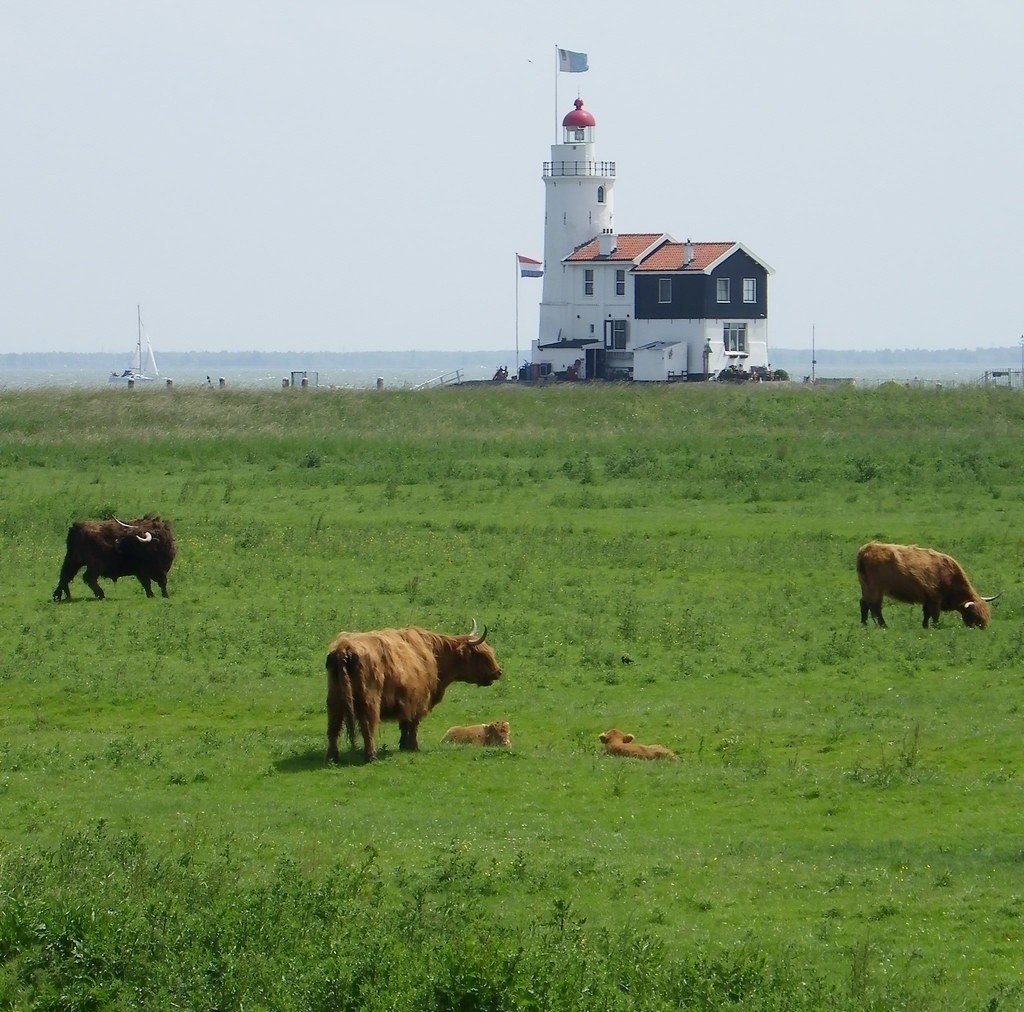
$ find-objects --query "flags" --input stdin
[555,48,589,72]
[516,255,544,278]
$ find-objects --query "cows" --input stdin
[597,729,679,760]
[857,540,1004,631]
[325,617,504,765]
[52,513,176,603]
[441,721,513,750]
